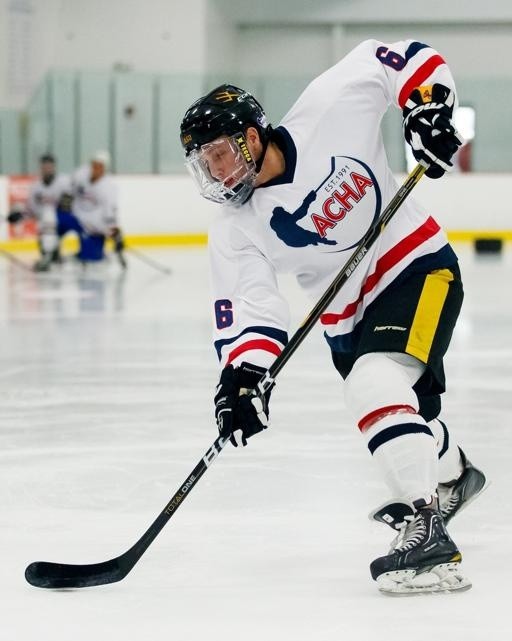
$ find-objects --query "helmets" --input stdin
[180,84,271,209]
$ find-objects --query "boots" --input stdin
[435,445,488,526]
[371,495,462,581]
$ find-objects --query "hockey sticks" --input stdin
[25,157,431,589]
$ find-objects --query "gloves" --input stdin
[402,84,465,178]
[214,362,276,447]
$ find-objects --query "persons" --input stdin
[26,152,74,272]
[58,150,128,271]
[178,37,488,583]
